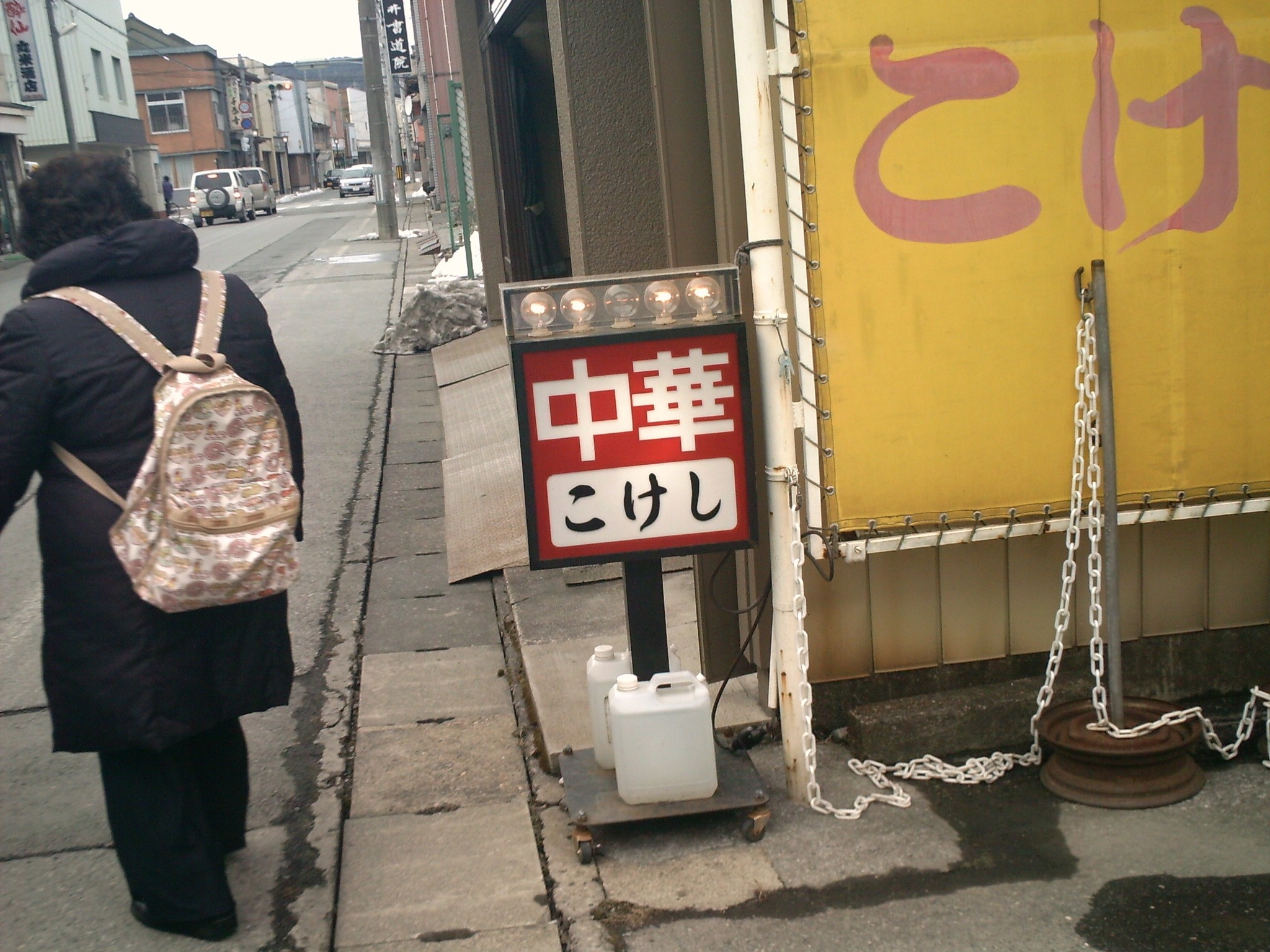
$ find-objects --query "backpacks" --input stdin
[19,261,299,612]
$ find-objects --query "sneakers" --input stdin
[127,895,239,943]
[217,830,247,854]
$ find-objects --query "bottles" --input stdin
[585,644,683,770]
[607,670,719,803]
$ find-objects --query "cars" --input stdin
[338,168,375,198]
[351,165,375,193]
[323,169,345,188]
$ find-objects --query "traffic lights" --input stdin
[268,82,295,90]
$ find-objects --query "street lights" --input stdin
[282,133,294,194]
[333,137,340,169]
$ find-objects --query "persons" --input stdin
[161,175,174,216]
[0,150,305,942]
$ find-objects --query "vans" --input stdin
[226,167,276,219]
[190,169,255,228]
